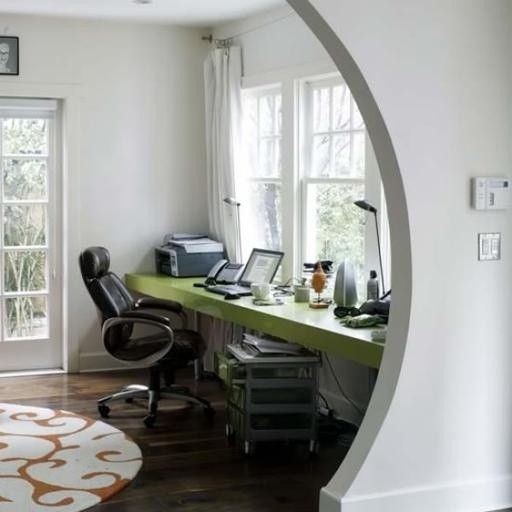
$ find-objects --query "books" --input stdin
[224,331,320,368]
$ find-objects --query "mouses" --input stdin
[225,294,240,299]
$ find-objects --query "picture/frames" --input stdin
[0,36,20,76]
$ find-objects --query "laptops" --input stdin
[205,248,284,296]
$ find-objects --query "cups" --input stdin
[250,282,270,301]
[294,284,309,303]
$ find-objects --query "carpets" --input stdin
[1,402,144,511]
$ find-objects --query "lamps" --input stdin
[224,196,243,264]
[354,200,386,297]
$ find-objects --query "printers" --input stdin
[155,238,224,277]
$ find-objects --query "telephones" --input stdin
[205,259,247,286]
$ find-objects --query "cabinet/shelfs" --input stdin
[222,339,322,459]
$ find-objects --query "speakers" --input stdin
[334,260,357,307]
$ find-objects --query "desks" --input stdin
[124,268,384,383]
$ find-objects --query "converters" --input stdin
[320,407,333,416]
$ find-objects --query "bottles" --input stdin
[367,268,381,303]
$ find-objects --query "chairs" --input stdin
[79,244,219,429]
